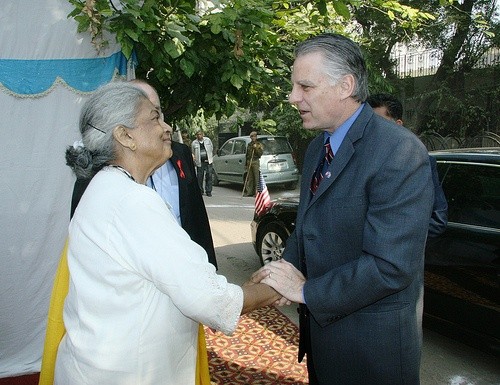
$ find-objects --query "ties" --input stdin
[309,137,335,195]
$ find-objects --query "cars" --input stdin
[212,135,300,196]
[249,147,500,351]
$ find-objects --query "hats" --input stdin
[249,131,257,138]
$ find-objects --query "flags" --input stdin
[256,170,270,216]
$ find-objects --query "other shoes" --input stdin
[200,192,203,195]
[207,191,212,197]
[243,194,253,197]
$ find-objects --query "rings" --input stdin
[269,272,273,278]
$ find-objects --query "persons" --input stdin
[367,93,448,244]
[191,130,213,197]
[252,33,436,385]
[70,79,219,273]
[53,83,282,385]
[181,129,191,146]
[242,131,264,198]
